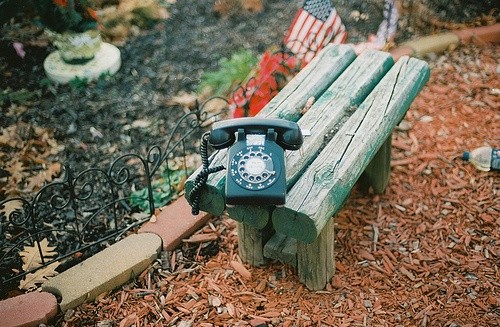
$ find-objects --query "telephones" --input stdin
[208,117,304,206]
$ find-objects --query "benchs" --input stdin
[183,40,430,294]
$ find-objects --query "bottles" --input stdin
[461,146,500,173]
